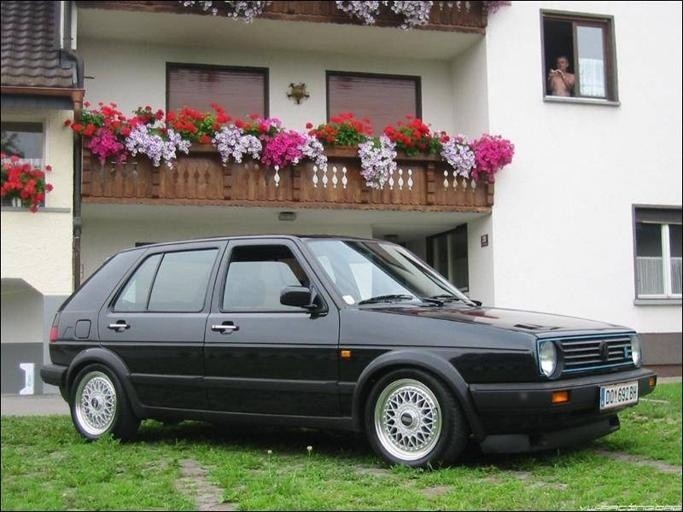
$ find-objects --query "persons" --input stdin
[546,56,575,98]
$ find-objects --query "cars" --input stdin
[39,232,657,470]
